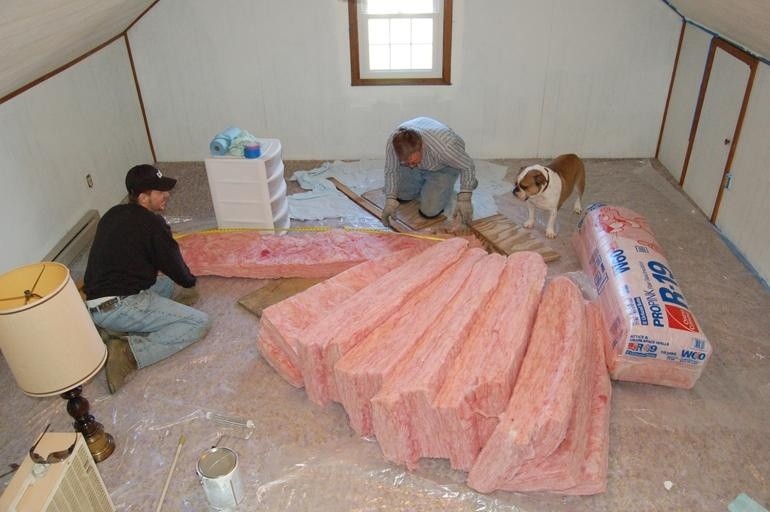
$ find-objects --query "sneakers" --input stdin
[96,324,138,395]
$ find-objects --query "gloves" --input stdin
[382,197,400,228]
[453,191,473,224]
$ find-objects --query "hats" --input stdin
[125,164,177,192]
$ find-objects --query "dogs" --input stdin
[512,153,587,240]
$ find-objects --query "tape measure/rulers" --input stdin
[171,227,447,241]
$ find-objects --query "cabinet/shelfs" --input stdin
[202,137,292,239]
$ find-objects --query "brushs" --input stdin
[196,412,255,475]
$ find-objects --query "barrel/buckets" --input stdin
[197,446,245,511]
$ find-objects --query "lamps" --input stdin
[0,262,116,465]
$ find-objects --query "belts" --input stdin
[85,297,128,314]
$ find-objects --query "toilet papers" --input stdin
[210,126,243,154]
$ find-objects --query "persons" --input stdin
[382,117,477,233]
[83,165,211,393]
